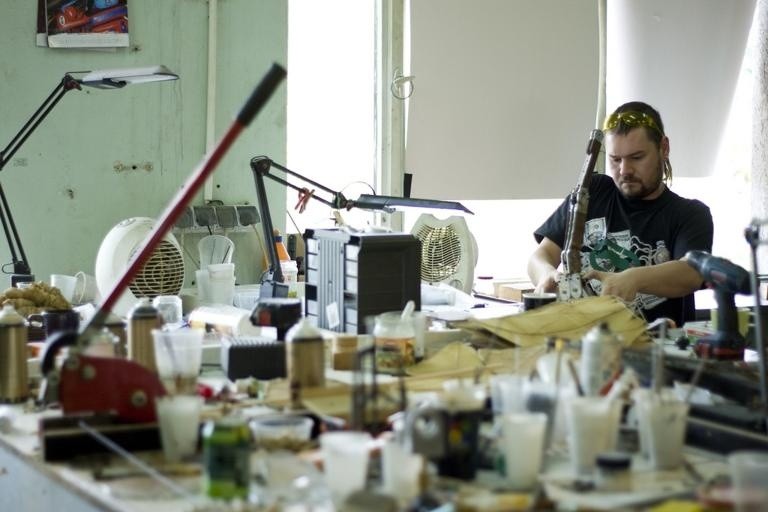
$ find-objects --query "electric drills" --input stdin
[685,250,760,361]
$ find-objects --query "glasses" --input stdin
[604,109,665,137]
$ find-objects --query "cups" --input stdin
[372,311,429,371]
[248,335,692,512]
[148,232,238,470]
[711,304,750,341]
[731,449,766,511]
[251,288,318,363]
[41,258,127,331]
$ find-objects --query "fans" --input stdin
[407,211,482,298]
[92,215,187,319]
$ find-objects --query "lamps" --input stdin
[0,63,180,290]
[248,150,480,299]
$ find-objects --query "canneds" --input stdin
[202,415,258,501]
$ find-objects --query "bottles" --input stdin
[578,321,627,411]
[1,295,31,411]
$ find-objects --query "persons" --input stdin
[528,101,714,323]
